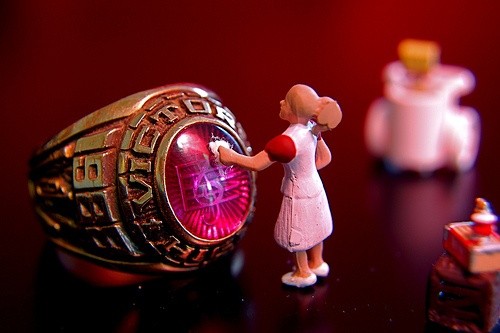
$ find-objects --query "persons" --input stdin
[221,84,344,288]
